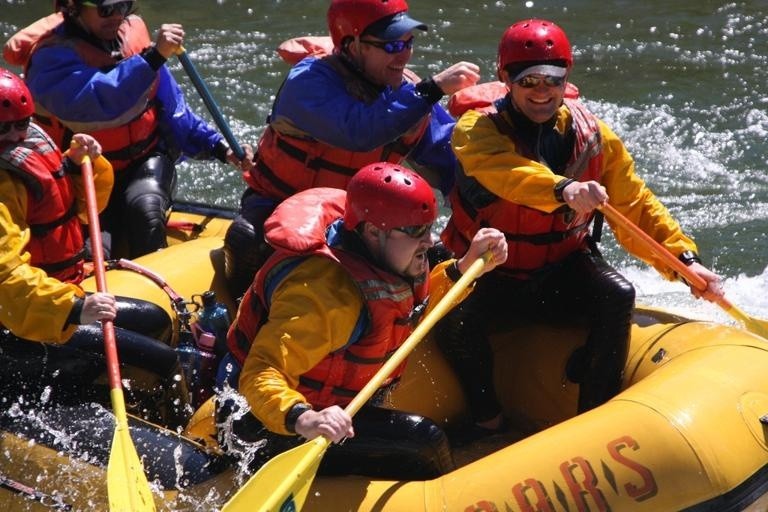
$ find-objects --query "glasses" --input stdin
[393,222,434,239]
[518,76,564,88]
[360,35,414,53]
[0,117,30,135]
[97,2,128,18]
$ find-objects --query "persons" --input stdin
[1,68,193,435]
[433,20,725,452]
[223,1,482,323]
[214,161,508,483]
[24,0,254,270]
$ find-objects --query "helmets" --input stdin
[496,19,573,72]
[0,67,34,122]
[54,0,64,13]
[343,161,439,266]
[328,0,408,72]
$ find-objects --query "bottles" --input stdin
[192,291,233,363]
[174,321,196,389]
[191,322,218,410]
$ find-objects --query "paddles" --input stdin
[176,45,245,161]
[598,202,767,341]
[79,145,157,512]
[219,250,492,512]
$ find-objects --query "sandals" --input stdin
[444,418,507,446]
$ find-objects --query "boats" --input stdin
[0,212,768,512]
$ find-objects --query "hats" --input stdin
[504,60,569,83]
[365,12,428,41]
